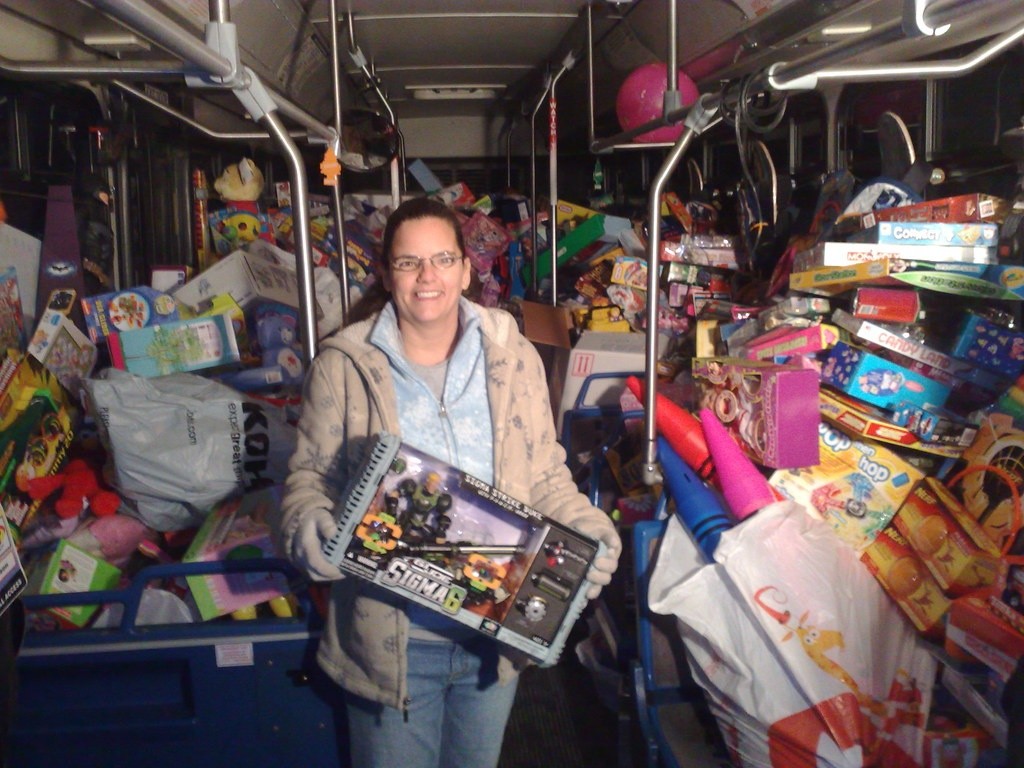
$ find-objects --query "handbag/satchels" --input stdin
[78,367,297,531]
[646,489,937,768]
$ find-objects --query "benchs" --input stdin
[0,555,354,768]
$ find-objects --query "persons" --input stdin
[279,199,621,767]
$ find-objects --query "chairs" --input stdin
[563,371,736,768]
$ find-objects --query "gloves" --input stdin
[586,533,623,599]
[291,507,346,581]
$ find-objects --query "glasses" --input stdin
[388,251,463,271]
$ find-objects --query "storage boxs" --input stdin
[321,431,608,668]
[0,179,1024,751]
